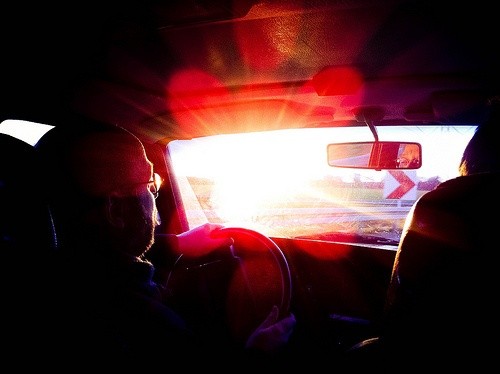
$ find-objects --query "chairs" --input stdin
[0,133,134,374]
[333,170,500,374]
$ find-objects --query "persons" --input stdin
[458,105,500,175]
[34,119,296,374]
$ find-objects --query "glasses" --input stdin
[400,162,405,164]
[105,171,163,201]
